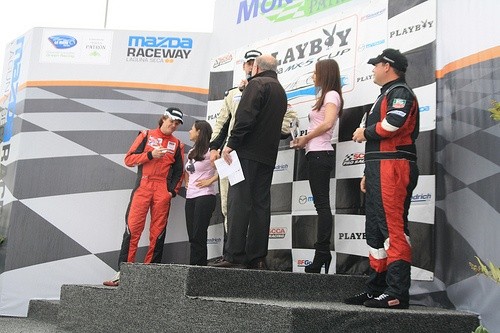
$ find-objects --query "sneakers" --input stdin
[363,294,410,310]
[344,291,380,304]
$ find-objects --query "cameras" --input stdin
[187,164,195,174]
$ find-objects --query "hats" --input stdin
[164,107,184,124]
[367,49,408,73]
[243,49,262,63]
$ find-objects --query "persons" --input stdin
[293,59,344,274]
[103,107,185,286]
[184,120,219,266]
[210,50,300,270]
[345,48,419,309]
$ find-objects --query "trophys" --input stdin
[357,112,368,143]
[289,117,306,148]
[150,138,164,160]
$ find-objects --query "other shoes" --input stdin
[215,259,247,269]
[248,259,266,270]
[103,272,120,286]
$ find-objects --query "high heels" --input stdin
[304,253,331,275]
